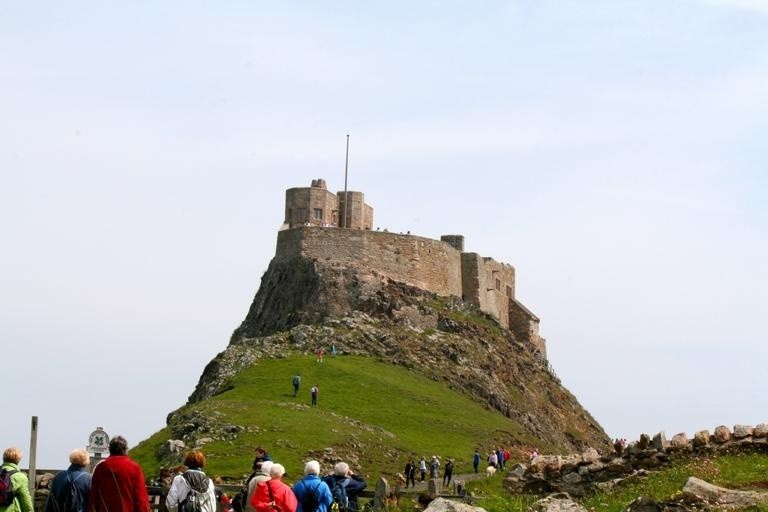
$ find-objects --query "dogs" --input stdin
[486,466,498,477]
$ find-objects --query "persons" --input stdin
[158,450,368,511]
[310,383,320,406]
[404,452,455,488]
[316,349,324,367]
[292,371,301,396]
[89,435,154,510]
[303,219,413,235]
[487,445,511,470]
[45,449,97,510]
[473,450,481,473]
[611,438,628,448]
[329,339,337,359]
[0,446,35,512]
[530,447,539,459]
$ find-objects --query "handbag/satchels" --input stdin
[232,485,248,512]
[178,488,202,512]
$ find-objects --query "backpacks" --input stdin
[57,470,87,511]
[0,465,20,507]
[330,478,351,512]
[301,479,324,512]
